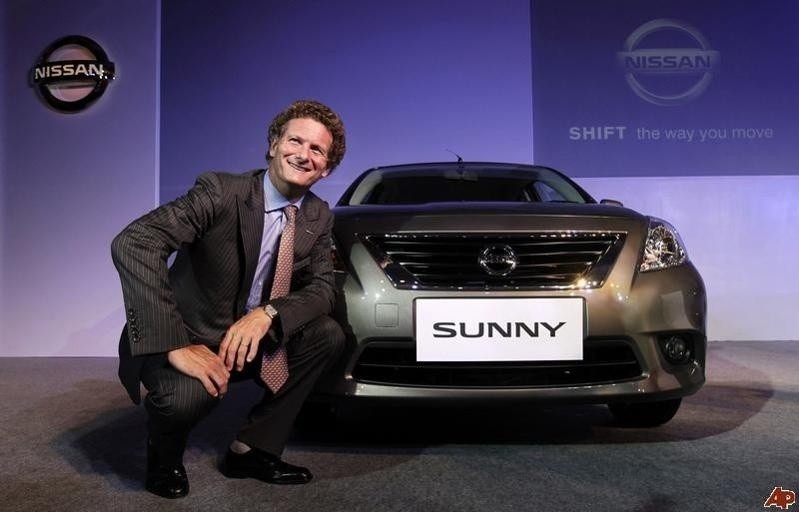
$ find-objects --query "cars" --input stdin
[323,148,707,446]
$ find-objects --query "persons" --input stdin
[107,97,346,498]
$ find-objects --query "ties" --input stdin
[260,204,292,393]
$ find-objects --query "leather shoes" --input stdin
[143,445,192,500]
[220,447,313,485]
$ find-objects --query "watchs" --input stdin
[264,302,278,319]
